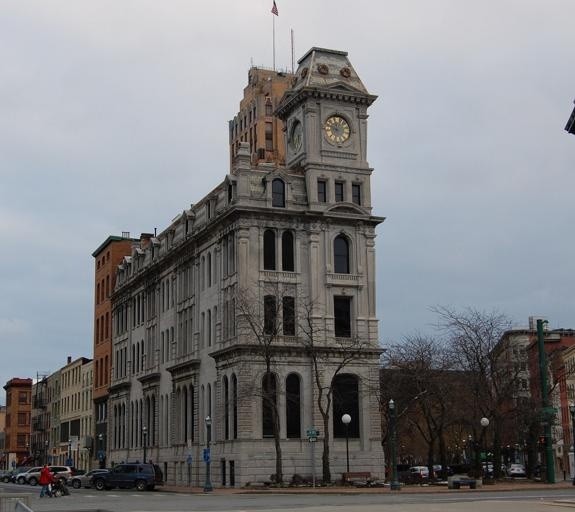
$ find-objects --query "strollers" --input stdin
[48,474,71,497]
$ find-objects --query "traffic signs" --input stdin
[309,437,318,442]
[307,430,320,437]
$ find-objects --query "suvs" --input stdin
[87,462,166,493]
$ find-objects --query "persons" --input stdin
[39,464,56,498]
[270,0,279,17]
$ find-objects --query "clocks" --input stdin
[321,111,354,148]
[288,119,305,153]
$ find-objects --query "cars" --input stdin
[406,460,530,483]
[66,468,115,490]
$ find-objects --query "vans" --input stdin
[0,466,85,487]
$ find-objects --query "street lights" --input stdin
[341,412,353,484]
[481,416,495,485]
[387,397,402,489]
[201,414,214,490]
[25,440,31,467]
[445,433,477,464]
[98,433,104,467]
[45,439,49,465]
[68,438,73,466]
[141,425,149,463]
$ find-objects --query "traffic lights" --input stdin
[539,436,548,446]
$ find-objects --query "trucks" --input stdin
[565,452,575,486]
